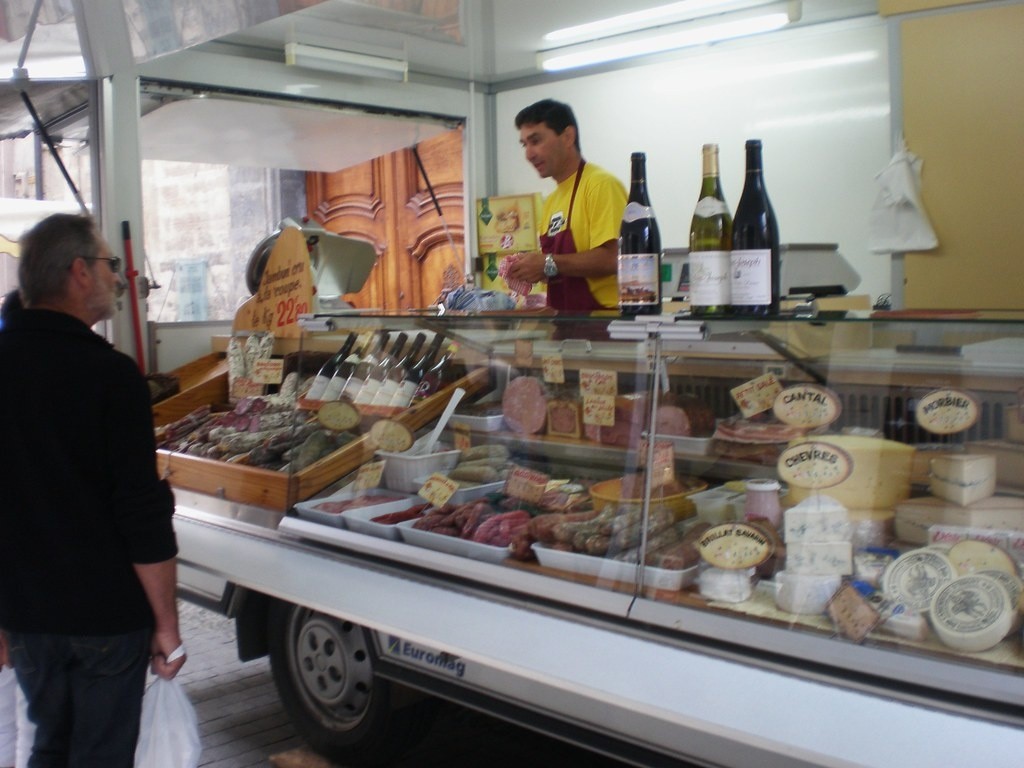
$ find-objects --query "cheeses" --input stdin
[782,434,1024,580]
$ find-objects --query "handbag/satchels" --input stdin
[132,644,202,768]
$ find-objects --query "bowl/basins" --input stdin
[591,471,709,521]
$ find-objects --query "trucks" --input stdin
[0,0,1024,768]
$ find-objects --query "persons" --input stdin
[0,212,187,768]
[508,100,629,317]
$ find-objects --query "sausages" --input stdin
[378,500,715,569]
[155,332,362,476]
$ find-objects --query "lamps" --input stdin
[532,3,803,74]
[283,40,409,84]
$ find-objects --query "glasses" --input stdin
[66,255,121,273]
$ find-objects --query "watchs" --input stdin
[543,253,558,278]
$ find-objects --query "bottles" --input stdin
[616,151,663,315]
[302,328,460,406]
[731,139,782,318]
[689,142,734,316]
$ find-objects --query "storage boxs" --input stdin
[477,192,546,253]
[150,337,1024,678]
[479,249,546,297]
